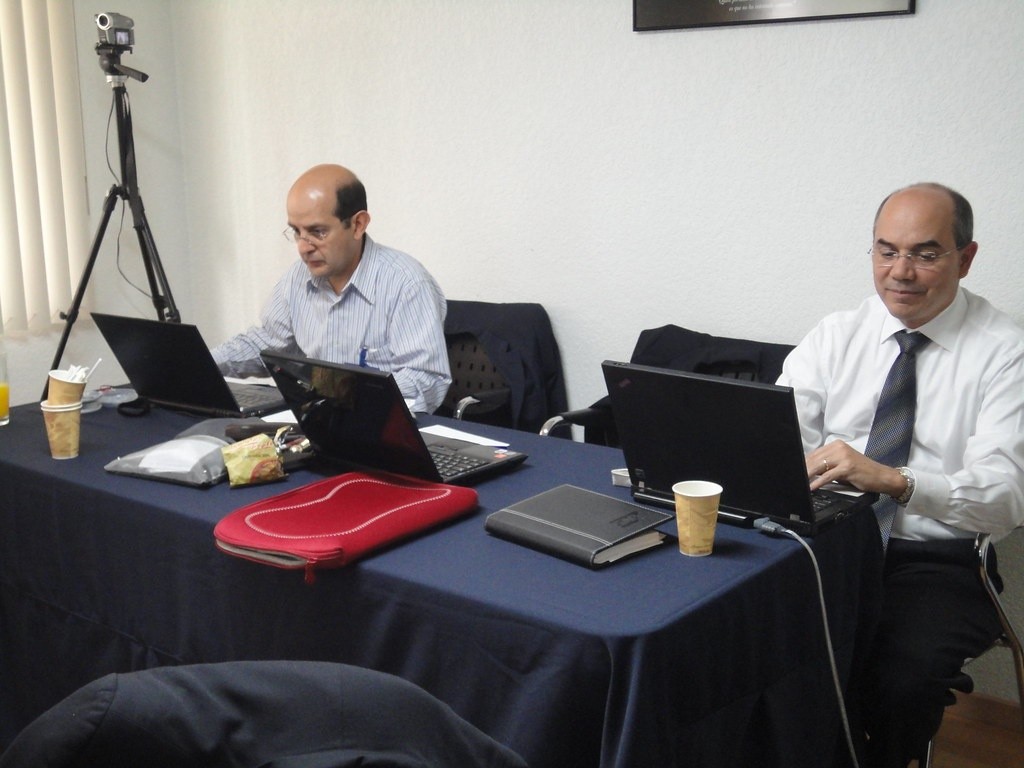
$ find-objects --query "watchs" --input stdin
[890,467,915,504]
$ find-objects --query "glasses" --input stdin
[282,215,351,246]
[866,247,960,268]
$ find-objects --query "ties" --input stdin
[854,329,932,560]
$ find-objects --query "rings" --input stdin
[824,459,829,471]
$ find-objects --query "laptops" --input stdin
[260,349,529,484]
[89,312,290,419]
[601,359,881,539]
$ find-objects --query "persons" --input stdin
[773,181,1024,768]
[206,164,452,416]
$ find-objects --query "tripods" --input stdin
[39,43,182,402]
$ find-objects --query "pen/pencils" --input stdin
[358,346,367,367]
[280,435,306,449]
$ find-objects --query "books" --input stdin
[485,485,674,570]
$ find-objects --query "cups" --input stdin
[1,357,10,426]
[40,370,87,460]
[672,480,722,557]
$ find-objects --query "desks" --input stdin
[0,383,886,768]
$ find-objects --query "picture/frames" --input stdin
[633,0,916,31]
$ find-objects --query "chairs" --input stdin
[432,301,797,449]
[914,531,1024,768]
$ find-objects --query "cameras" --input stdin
[94,12,135,46]
